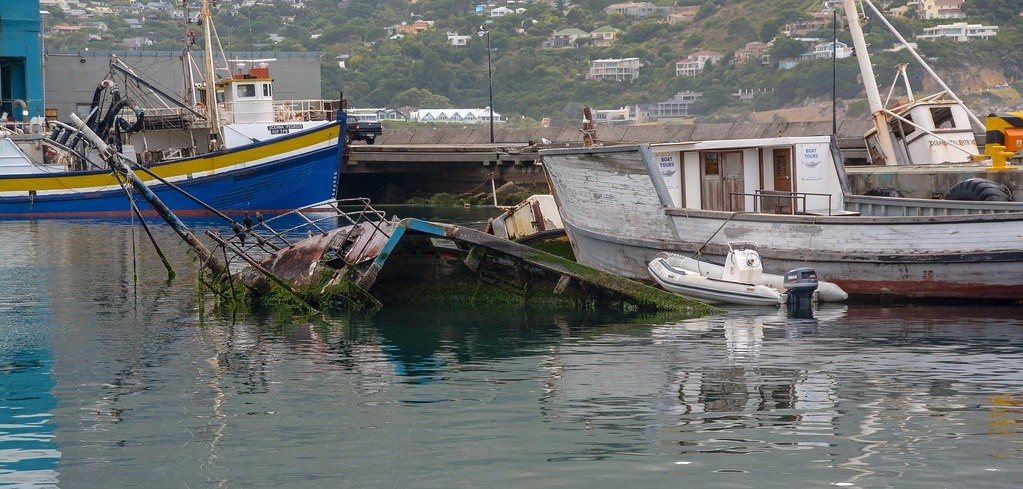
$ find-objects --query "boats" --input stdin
[538,1,1023,306]
[647,241,848,307]
[0,0,348,212]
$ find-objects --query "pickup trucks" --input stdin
[346,110,383,145]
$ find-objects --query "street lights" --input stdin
[822,8,837,137]
[478,24,494,143]
